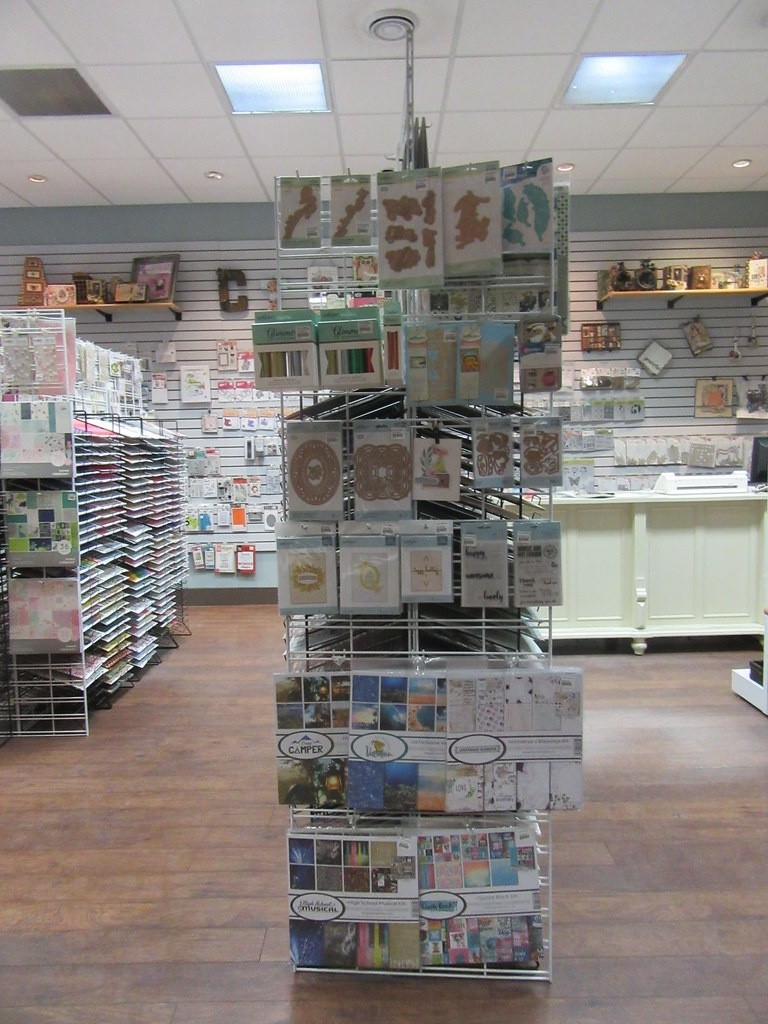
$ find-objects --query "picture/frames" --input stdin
[694,378,734,416]
[130,254,182,302]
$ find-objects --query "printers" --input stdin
[652,470,749,495]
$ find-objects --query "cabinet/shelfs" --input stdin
[258,148,577,982]
[0,394,186,735]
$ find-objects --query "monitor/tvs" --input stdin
[750,437,768,482]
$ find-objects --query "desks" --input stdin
[502,492,767,657]
